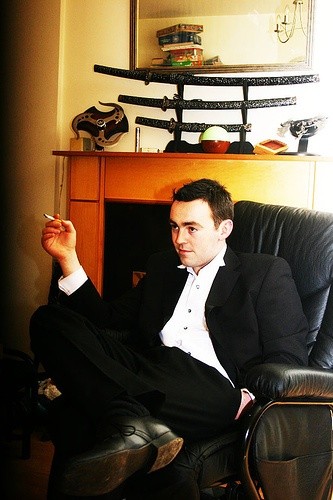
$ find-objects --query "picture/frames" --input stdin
[131,0,315,72]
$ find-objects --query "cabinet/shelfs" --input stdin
[52,150,323,299]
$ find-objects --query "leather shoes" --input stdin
[51,416,185,496]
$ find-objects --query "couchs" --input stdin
[36,200,332,499]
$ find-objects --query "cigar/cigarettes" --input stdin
[43,213,61,222]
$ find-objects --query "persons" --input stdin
[29,178,310,500]
